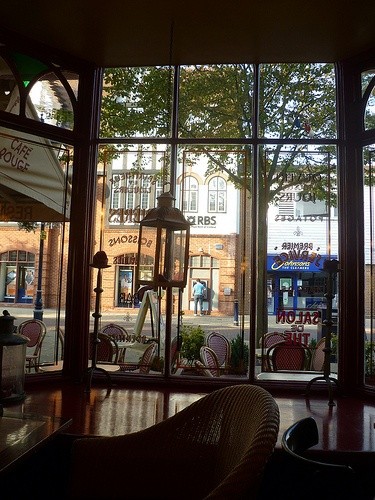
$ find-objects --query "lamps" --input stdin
[134,192,190,287]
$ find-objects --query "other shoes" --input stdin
[200,314,204,317]
[194,314,197,317]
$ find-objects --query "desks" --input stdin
[256,346,275,358]
[111,338,151,352]
[0,413,74,477]
[3,386,375,462]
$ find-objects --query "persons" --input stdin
[123,274,128,294]
[25,270,34,295]
[192,278,205,317]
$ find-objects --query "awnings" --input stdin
[0,80,70,222]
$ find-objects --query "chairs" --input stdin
[282,417,354,500]
[72,384,280,500]
[19,318,333,378]
